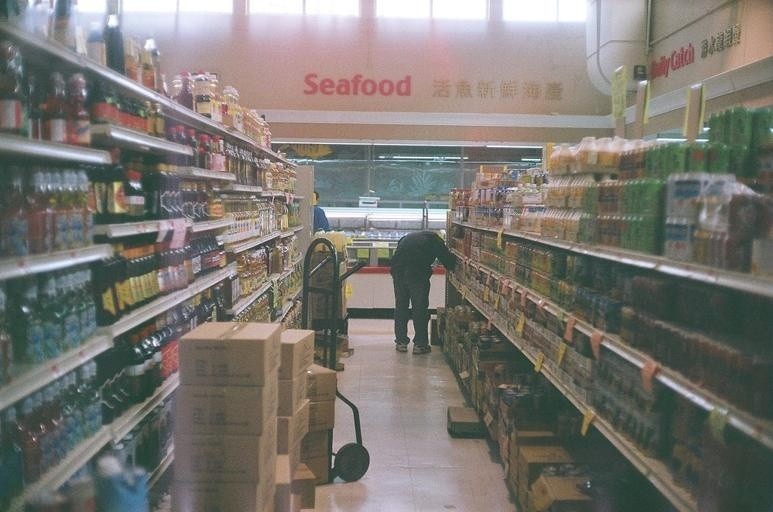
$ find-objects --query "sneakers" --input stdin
[396,344,408,352]
[413,344,431,354]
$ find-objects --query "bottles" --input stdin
[539,133,623,178]
[1,0,306,512]
[509,174,593,238]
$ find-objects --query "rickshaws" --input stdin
[301,237,371,486]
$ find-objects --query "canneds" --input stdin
[471,106,771,276]
[471,234,773,457]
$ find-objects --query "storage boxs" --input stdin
[170,321,337,512]
[498,399,595,512]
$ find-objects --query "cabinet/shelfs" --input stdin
[0,1,315,512]
[444,210,773,512]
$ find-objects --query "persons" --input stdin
[387,229,456,357]
[315,191,329,232]
